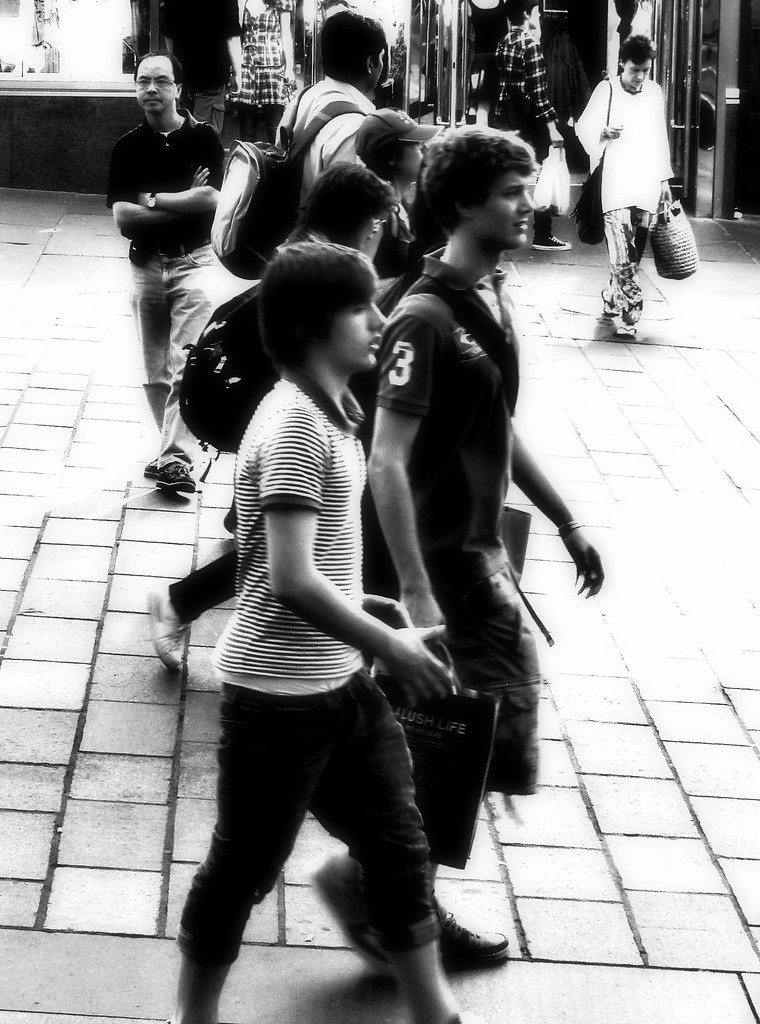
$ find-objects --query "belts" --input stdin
[149,238,211,256]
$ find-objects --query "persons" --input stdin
[176,242,467,1024]
[148,10,446,670]
[309,126,604,980]
[574,35,675,337]
[461,1,507,72]
[492,0,571,251]
[157,0,396,152]
[101,52,225,493]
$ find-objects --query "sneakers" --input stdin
[148,592,188,674]
[439,913,510,962]
[311,854,397,970]
[144,459,160,478]
[157,460,196,494]
[531,235,572,252]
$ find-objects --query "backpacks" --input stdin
[210,85,367,279]
[178,283,283,454]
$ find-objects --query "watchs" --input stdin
[147,192,158,208]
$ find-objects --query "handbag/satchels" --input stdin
[570,166,605,245]
[314,639,502,871]
[532,144,570,217]
[649,200,699,281]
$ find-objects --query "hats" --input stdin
[355,108,445,164]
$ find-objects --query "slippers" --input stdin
[617,324,638,338]
[135,78,181,88]
[602,289,620,317]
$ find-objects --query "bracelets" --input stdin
[558,520,579,538]
